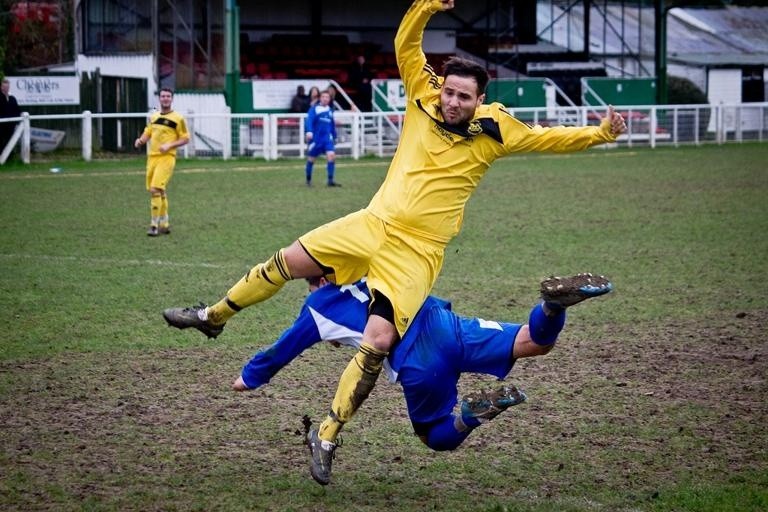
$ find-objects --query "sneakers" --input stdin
[328,182,342,187]
[147,223,170,237]
[305,428,343,487]
[161,300,227,340]
[539,272,614,307]
[460,385,528,423]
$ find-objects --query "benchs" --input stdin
[249,107,667,146]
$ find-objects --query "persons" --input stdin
[163,0,629,489]
[133,88,190,237]
[191,37,339,114]
[231,271,614,452]
[0,78,22,165]
[346,52,377,114]
[304,89,342,188]
